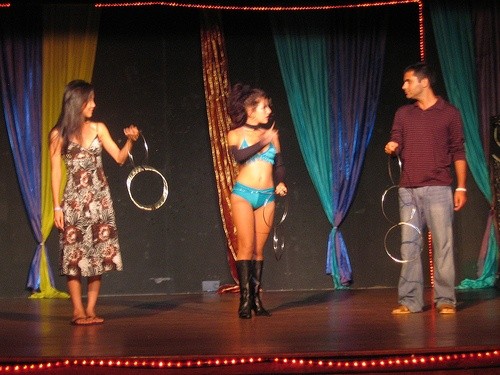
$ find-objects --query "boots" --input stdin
[236,260,253,318]
[250,260,271,316]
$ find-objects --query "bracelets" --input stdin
[455,187,466,191]
[54,207,61,211]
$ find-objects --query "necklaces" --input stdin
[244,122,258,130]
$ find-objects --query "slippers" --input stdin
[71,316,92,326]
[87,315,105,324]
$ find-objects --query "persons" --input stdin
[49,80,139,325]
[227,83,287,319]
[385,63,467,314]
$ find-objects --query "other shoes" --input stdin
[392,306,423,314]
[440,305,457,313]
